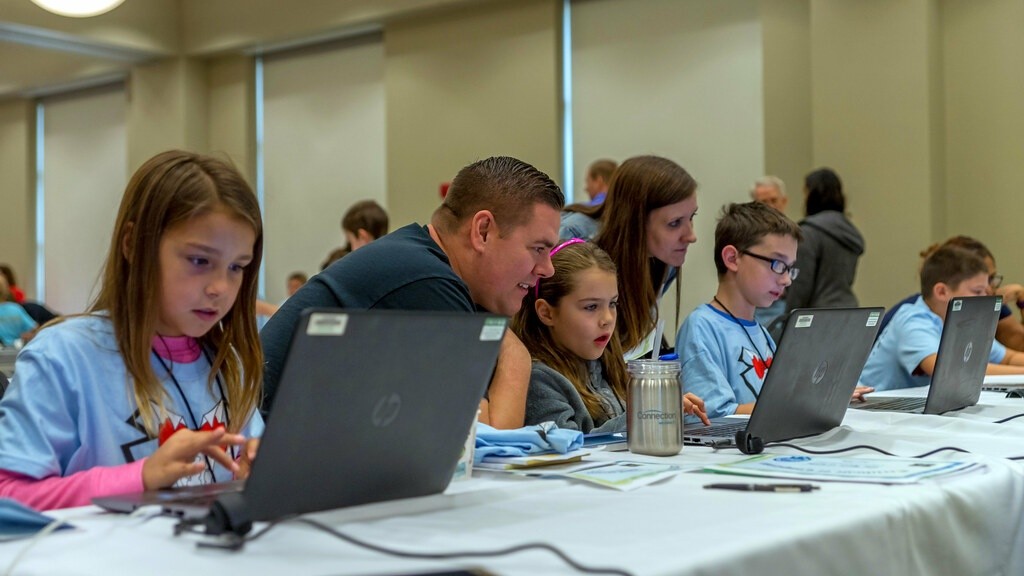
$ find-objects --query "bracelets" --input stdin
[1016,299,1024,310]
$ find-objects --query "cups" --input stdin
[626,358,684,454]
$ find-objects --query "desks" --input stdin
[1,374,1024,576]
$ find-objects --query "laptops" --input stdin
[981,381,1024,392]
[679,305,887,448]
[848,294,1004,415]
[93,308,514,530]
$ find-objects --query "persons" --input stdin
[0,263,63,346]
[257,199,390,317]
[509,238,710,434]
[256,157,566,430]
[674,202,874,424]
[855,234,1024,391]
[747,176,788,331]
[558,156,700,354]
[782,167,865,314]
[0,150,265,512]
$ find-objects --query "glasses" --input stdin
[738,247,800,281]
[988,275,1003,288]
